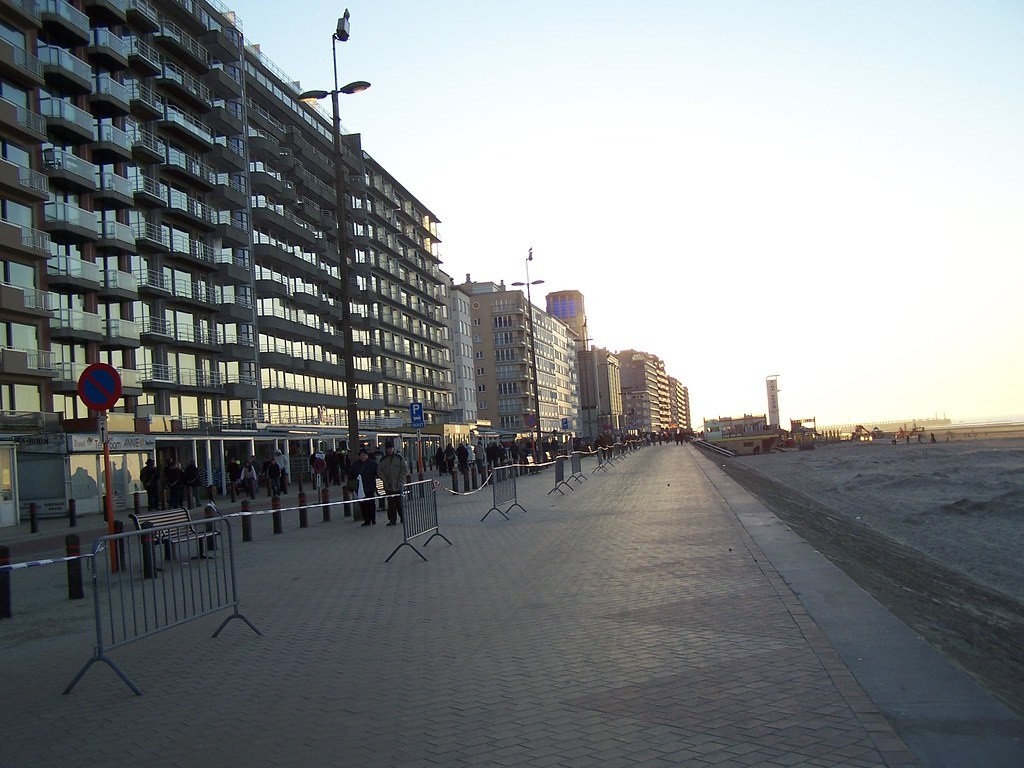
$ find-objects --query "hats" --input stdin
[386,442,394,448]
[275,449,282,454]
[145,459,154,464]
[358,449,368,456]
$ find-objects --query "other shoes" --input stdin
[400,518,403,523]
[386,522,396,527]
[153,507,161,511]
[284,492,288,494]
[277,493,280,496]
[148,506,152,512]
[197,504,201,507]
[250,497,256,500]
[372,520,376,525]
[361,521,371,526]
[187,507,191,510]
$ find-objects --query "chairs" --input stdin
[44,149,61,170]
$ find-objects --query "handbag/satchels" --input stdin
[357,475,365,499]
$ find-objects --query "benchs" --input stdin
[129,506,221,571]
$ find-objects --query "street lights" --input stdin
[511,249,546,463]
[296,6,375,521]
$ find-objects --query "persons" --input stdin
[226,458,240,496]
[140,458,202,511]
[906,435,909,444]
[261,449,288,499]
[310,446,384,490]
[376,442,406,526]
[102,461,131,494]
[593,431,704,460]
[434,438,560,476]
[72,467,96,499]
[918,434,922,444]
[240,456,259,498]
[347,449,377,526]
